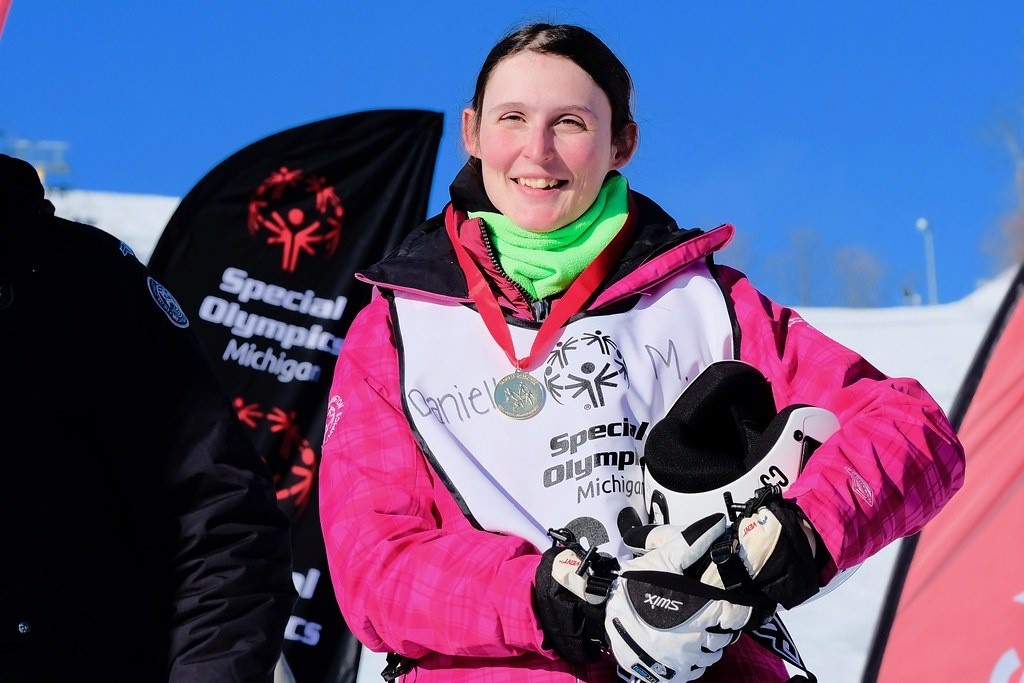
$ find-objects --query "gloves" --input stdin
[623,485,832,612]
[535,512,777,683]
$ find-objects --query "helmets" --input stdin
[644,360,840,525]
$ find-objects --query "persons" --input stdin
[319,23,966,683]
[0,154,297,683]
